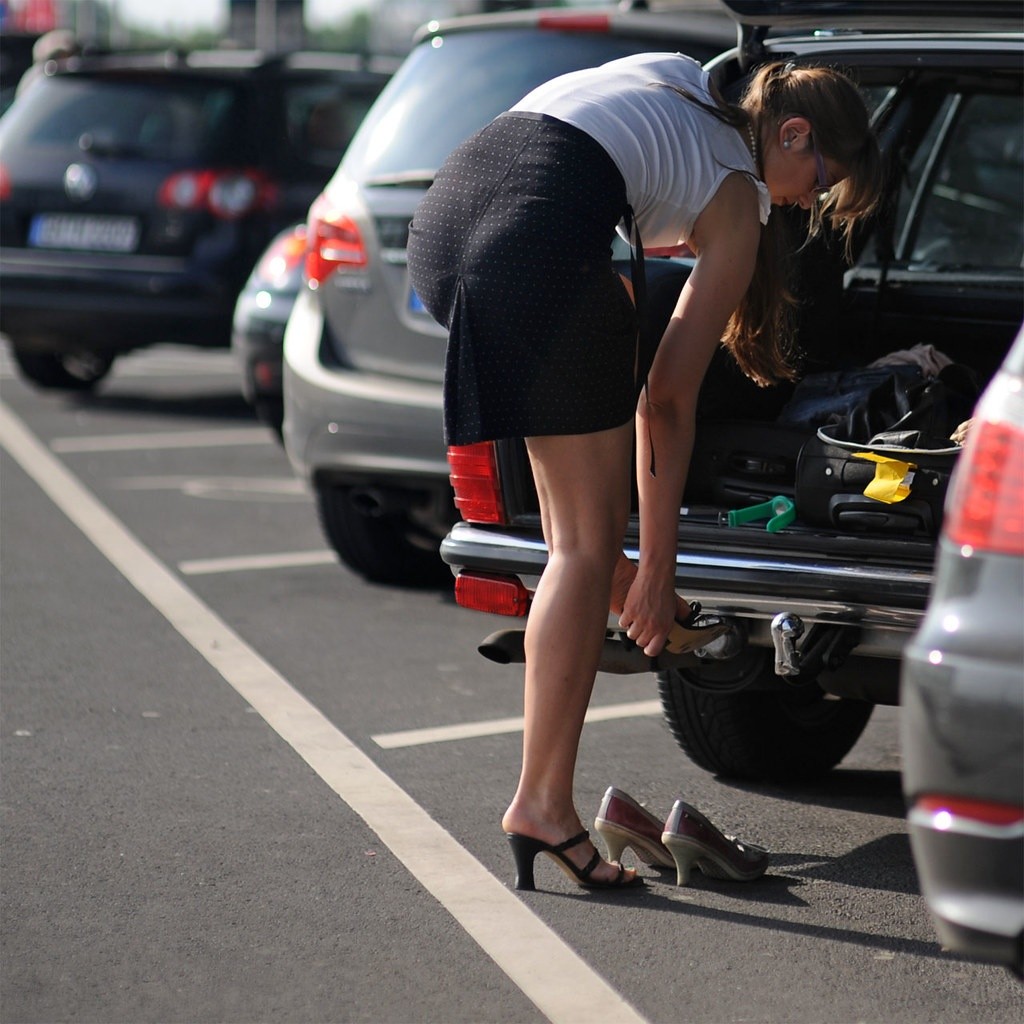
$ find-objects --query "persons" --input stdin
[405,53,886,888]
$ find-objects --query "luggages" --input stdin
[796,424,966,536]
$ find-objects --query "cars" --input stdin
[226,222,308,453]
[436,0,1023,793]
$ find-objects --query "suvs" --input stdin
[284,2,815,598]
[0,28,406,403]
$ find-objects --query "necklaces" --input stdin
[747,122,757,163]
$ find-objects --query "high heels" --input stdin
[663,800,768,887]
[595,786,701,871]
[623,599,731,657]
[505,827,644,892]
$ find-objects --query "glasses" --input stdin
[810,132,833,203]
